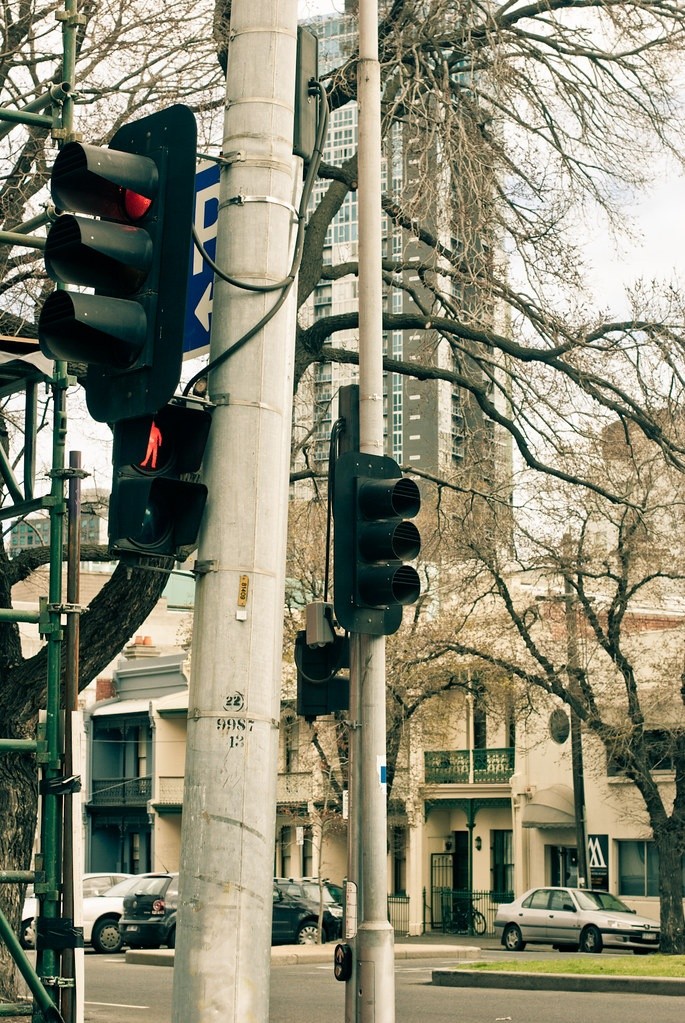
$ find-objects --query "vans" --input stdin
[273,876,344,940]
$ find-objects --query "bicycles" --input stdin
[444,897,487,935]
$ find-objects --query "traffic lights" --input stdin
[105,395,215,557]
[332,449,421,633]
[35,104,197,424]
[295,629,351,719]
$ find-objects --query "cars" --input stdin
[73,872,179,954]
[19,871,136,950]
[118,871,335,947]
[493,886,661,954]
